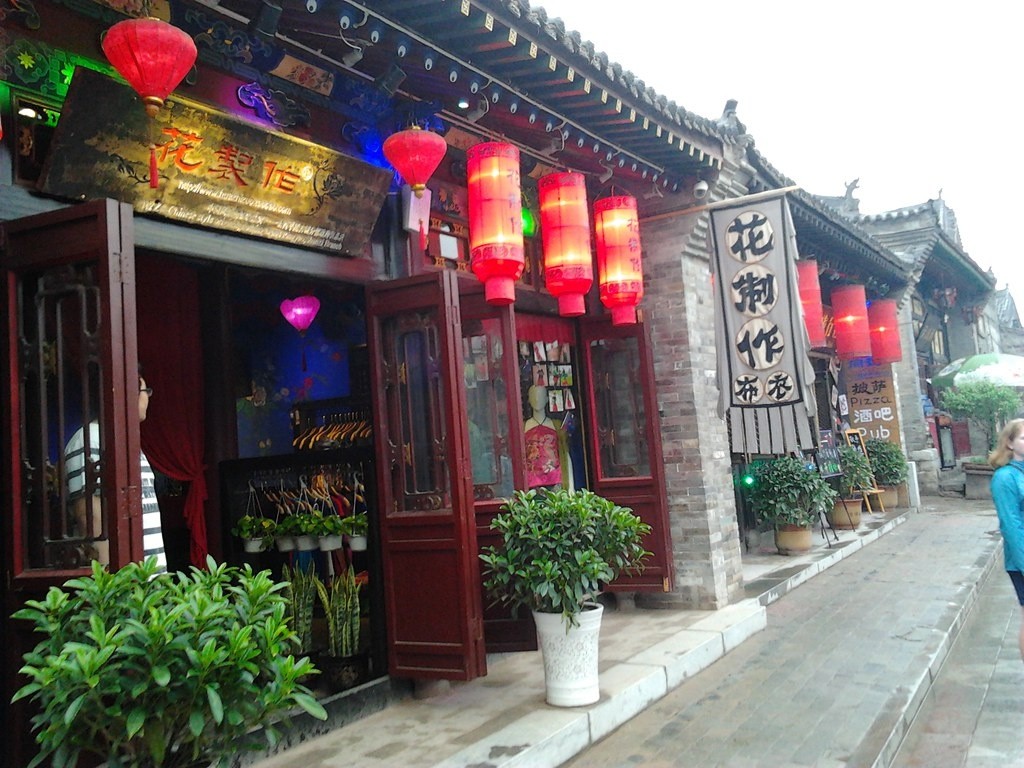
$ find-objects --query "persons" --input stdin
[524,385,575,499]
[988,419,1024,660]
[64,375,167,582]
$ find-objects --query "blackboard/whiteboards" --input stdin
[814,429,844,479]
[844,429,879,494]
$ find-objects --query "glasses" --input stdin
[137,388,153,398]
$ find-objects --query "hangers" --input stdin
[262,461,365,515]
[292,411,373,451]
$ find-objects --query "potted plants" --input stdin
[749,453,836,556]
[334,512,366,551]
[276,557,319,694]
[478,488,654,708]
[939,375,1020,499]
[315,562,367,690]
[313,509,343,551]
[275,522,296,552]
[292,515,320,551]
[823,447,875,528]
[11,554,328,768]
[866,438,910,508]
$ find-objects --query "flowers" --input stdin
[234,514,274,552]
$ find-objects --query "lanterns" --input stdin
[593,196,643,325]
[796,260,827,349]
[102,17,197,188]
[538,172,593,316]
[868,298,902,365]
[831,284,872,360]
[382,126,447,251]
[466,141,525,305]
[280,295,321,371]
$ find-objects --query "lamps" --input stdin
[248,0,283,43]
[373,64,407,98]
[341,48,364,69]
[458,96,472,110]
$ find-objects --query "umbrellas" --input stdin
[932,352,1024,387]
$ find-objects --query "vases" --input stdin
[245,537,267,552]
